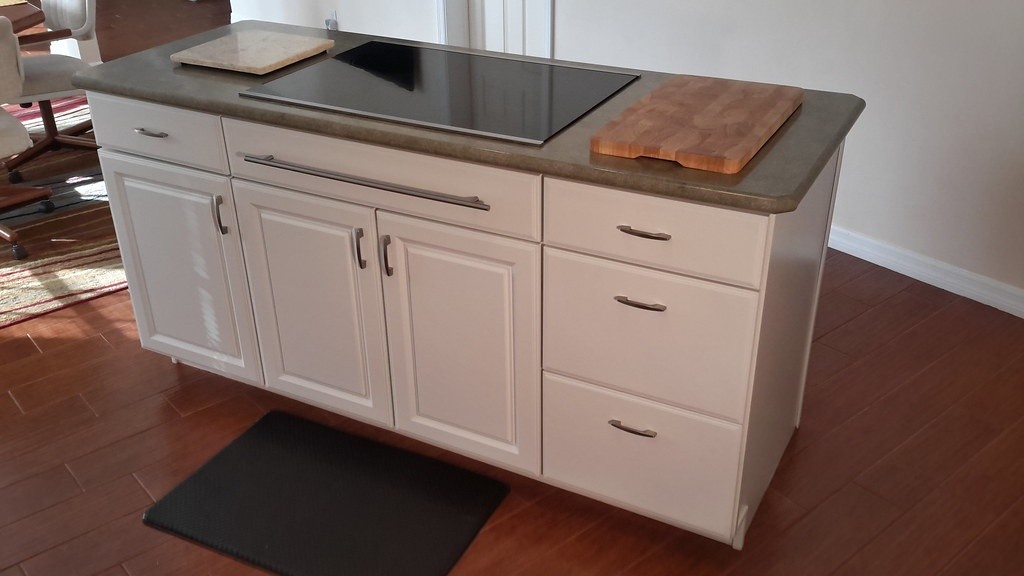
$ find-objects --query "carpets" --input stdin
[142,408,512,576]
[0,94,129,330]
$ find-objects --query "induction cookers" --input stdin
[240,39,640,146]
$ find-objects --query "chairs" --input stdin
[0,0,103,183]
[0,15,54,260]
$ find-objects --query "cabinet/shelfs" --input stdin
[543,136,845,550]
[85,88,265,389]
[220,112,543,481]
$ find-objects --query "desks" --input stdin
[0,0,45,34]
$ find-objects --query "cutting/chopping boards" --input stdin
[170,28,335,76]
[591,76,806,174]
